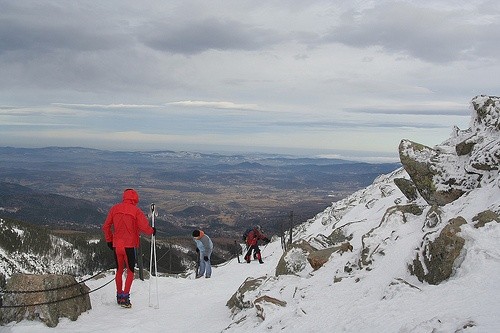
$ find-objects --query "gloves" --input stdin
[196,247,199,252]
[107,242,113,249]
[153,227,156,235]
[203,256,209,261]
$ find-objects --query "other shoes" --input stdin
[196,274,202,278]
[206,274,211,278]
[247,260,250,263]
[259,260,264,264]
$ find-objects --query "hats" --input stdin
[192,230,200,237]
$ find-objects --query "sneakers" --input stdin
[117,292,123,304]
[121,294,132,308]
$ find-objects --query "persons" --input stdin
[245,224,270,264]
[103,189,156,308]
[192,230,214,278]
